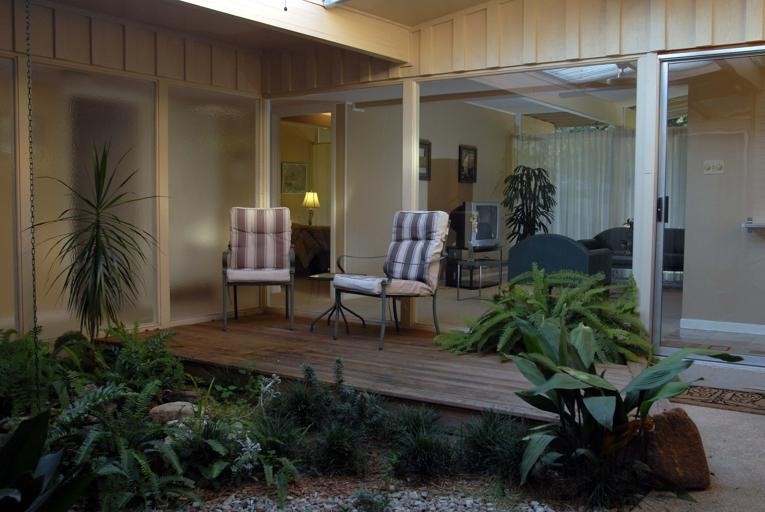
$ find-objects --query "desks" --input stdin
[291,223,330,280]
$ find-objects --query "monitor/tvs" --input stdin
[449,201,504,251]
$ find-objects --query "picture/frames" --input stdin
[458,144,477,184]
[282,161,308,193]
[418,138,432,181]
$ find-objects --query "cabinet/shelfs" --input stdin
[445,244,504,289]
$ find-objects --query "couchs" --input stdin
[595,225,684,287]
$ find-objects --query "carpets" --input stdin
[667,384,765,415]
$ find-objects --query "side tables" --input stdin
[308,272,367,341]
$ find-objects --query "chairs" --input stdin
[507,234,611,298]
[334,209,450,351]
[222,205,298,331]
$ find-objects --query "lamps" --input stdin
[299,191,321,226]
